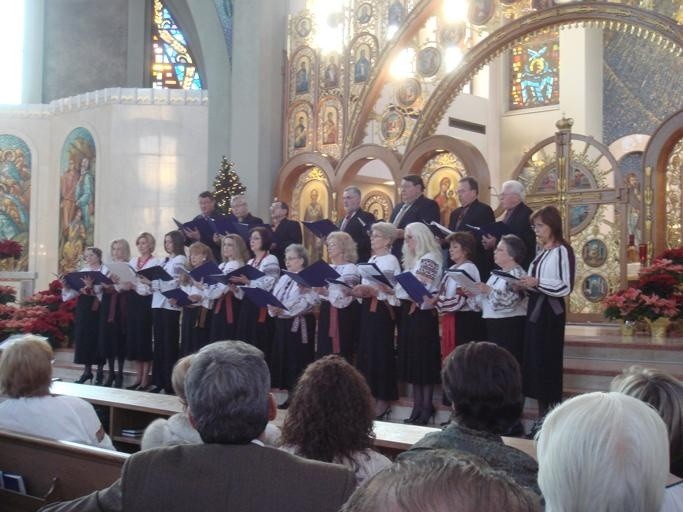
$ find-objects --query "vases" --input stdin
[643,313,675,338]
[615,315,641,337]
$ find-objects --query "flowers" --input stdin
[1,240,76,350]
[606,242,682,323]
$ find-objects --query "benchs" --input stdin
[0,374,682,509]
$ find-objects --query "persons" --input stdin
[58,175,575,438]
[40,341,683,512]
[0,333,117,451]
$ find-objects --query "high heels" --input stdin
[75,373,93,384]
[405,406,436,425]
[94,369,123,387]
[374,406,391,420]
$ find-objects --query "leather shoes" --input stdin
[126,382,173,395]
[277,400,291,409]
